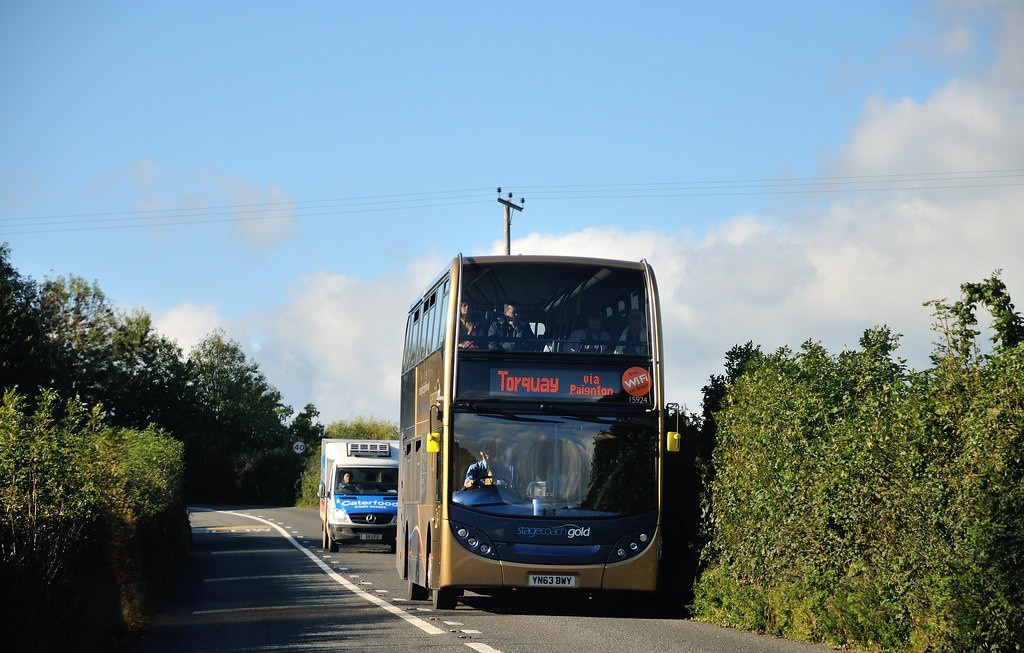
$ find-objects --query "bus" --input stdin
[395,253,684,609]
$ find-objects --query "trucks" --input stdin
[317,437,399,554]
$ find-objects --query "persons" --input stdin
[337,471,359,494]
[488,303,537,351]
[614,308,648,354]
[565,312,612,353]
[458,299,478,349]
[464,440,513,487]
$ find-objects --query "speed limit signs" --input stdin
[292,440,306,454]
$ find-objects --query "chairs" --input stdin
[458,307,634,354]
